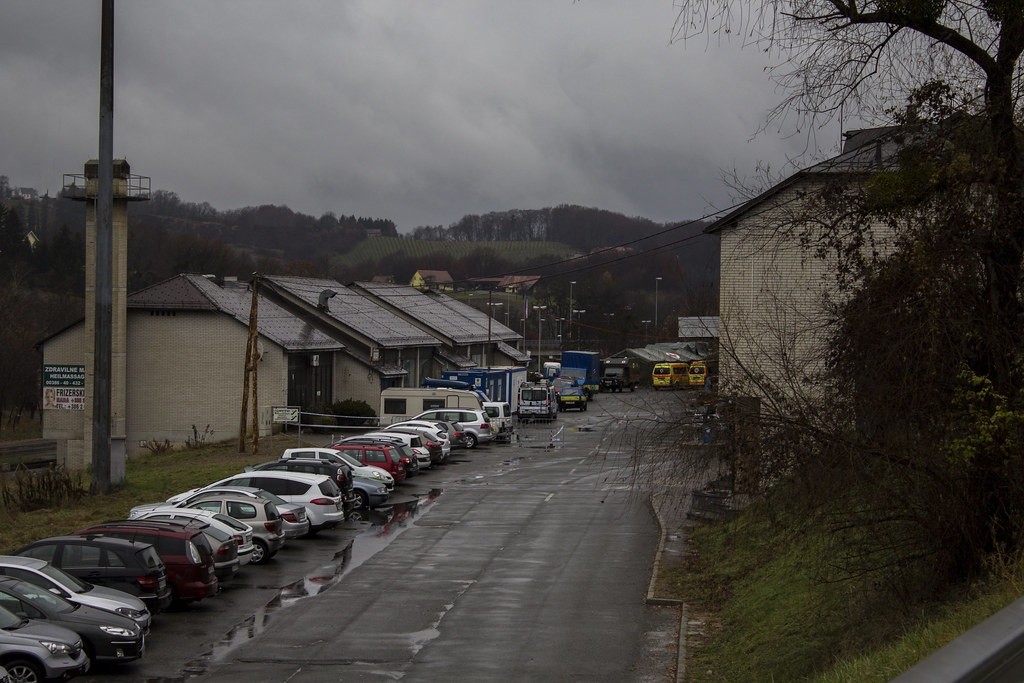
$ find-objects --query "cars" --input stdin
[281,448,395,491]
[559,387,588,412]
[131,486,312,536]
[484,401,513,432]
[10,535,172,616]
[553,378,579,393]
[184,496,286,564]
[0,555,152,637]
[0,575,145,662]
[384,420,449,438]
[165,470,345,538]
[129,509,255,567]
[374,428,443,467]
[427,420,468,448]
[0,606,91,683]
[101,518,239,583]
[365,433,431,469]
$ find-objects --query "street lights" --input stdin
[555,318,564,348]
[573,309,586,349]
[533,306,548,374]
[568,280,576,349]
[656,277,662,340]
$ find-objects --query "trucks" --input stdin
[560,351,600,401]
[600,356,642,393]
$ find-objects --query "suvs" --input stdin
[323,435,418,479]
[69,520,220,610]
[516,381,558,422]
[329,443,407,482]
[410,407,497,448]
[244,457,354,521]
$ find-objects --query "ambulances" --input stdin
[687,360,718,389]
[651,362,689,391]
